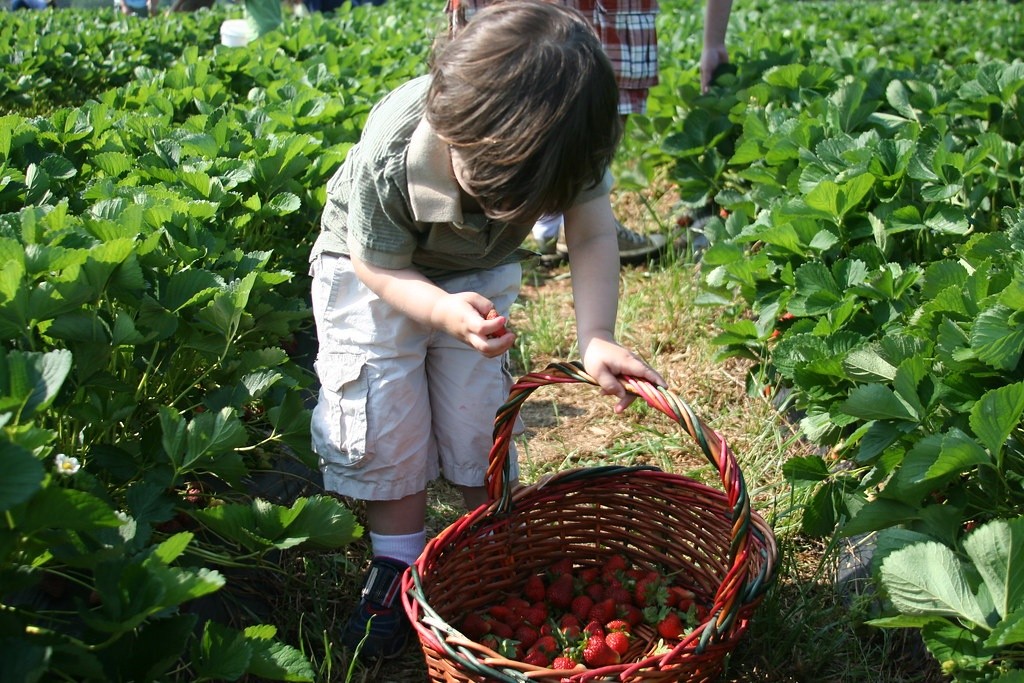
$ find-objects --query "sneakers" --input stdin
[556,221,667,258]
[340,555,413,660]
[535,237,563,261]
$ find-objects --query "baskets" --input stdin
[399,361,779,682]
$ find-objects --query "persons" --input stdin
[306,0,668,665]
[437,0,733,270]
[7,1,390,22]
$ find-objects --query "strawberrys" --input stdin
[720,210,728,219]
[186,486,225,510]
[464,555,712,675]
[763,312,794,398]
[676,214,692,228]
[485,309,507,338]
